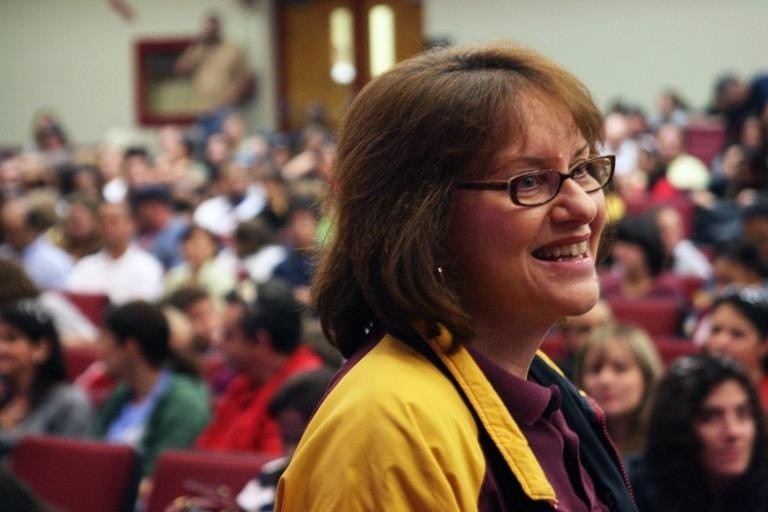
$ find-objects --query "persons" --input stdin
[274,40,637,512]
[1,14,359,511]
[556,76,768,512]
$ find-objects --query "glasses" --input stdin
[456,155,616,207]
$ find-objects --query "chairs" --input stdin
[8,436,269,511]
